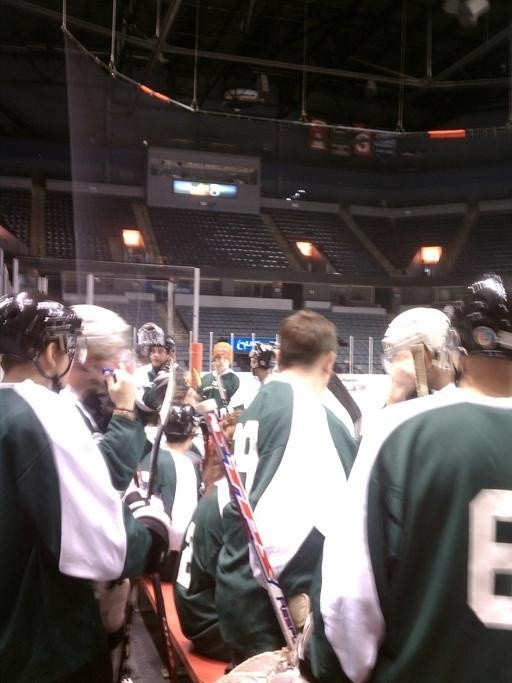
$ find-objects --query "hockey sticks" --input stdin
[327,374,362,444]
[117,360,175,683]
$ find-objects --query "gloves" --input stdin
[125,484,173,576]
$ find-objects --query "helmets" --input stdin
[163,404,196,436]
[377,305,453,374]
[249,342,277,369]
[1,288,84,359]
[452,269,512,359]
[212,341,234,361]
[148,332,177,356]
